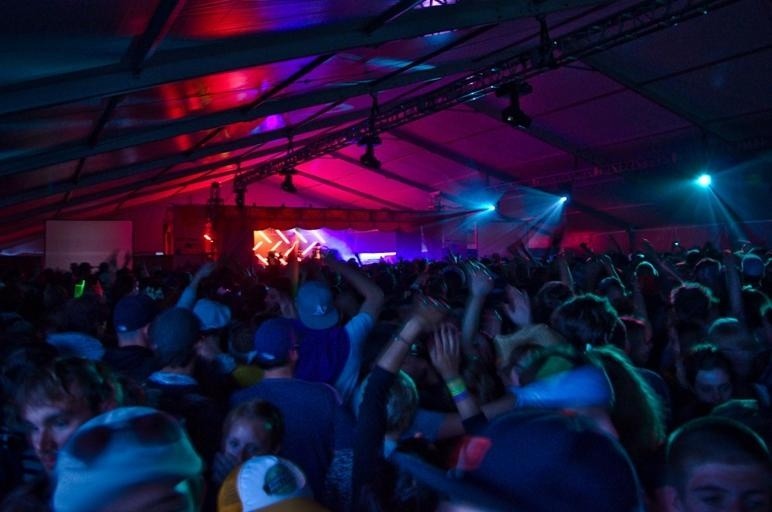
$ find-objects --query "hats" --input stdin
[352,368,419,433]
[255,317,304,363]
[54,404,209,512]
[113,295,157,333]
[217,453,313,512]
[397,406,644,512]
[150,307,202,352]
[296,280,339,330]
[741,254,764,277]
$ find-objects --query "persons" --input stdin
[1,223,772,511]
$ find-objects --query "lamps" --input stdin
[276,136,298,193]
[358,84,386,169]
[234,162,246,209]
[495,58,531,130]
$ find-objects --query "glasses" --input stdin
[71,412,181,458]
